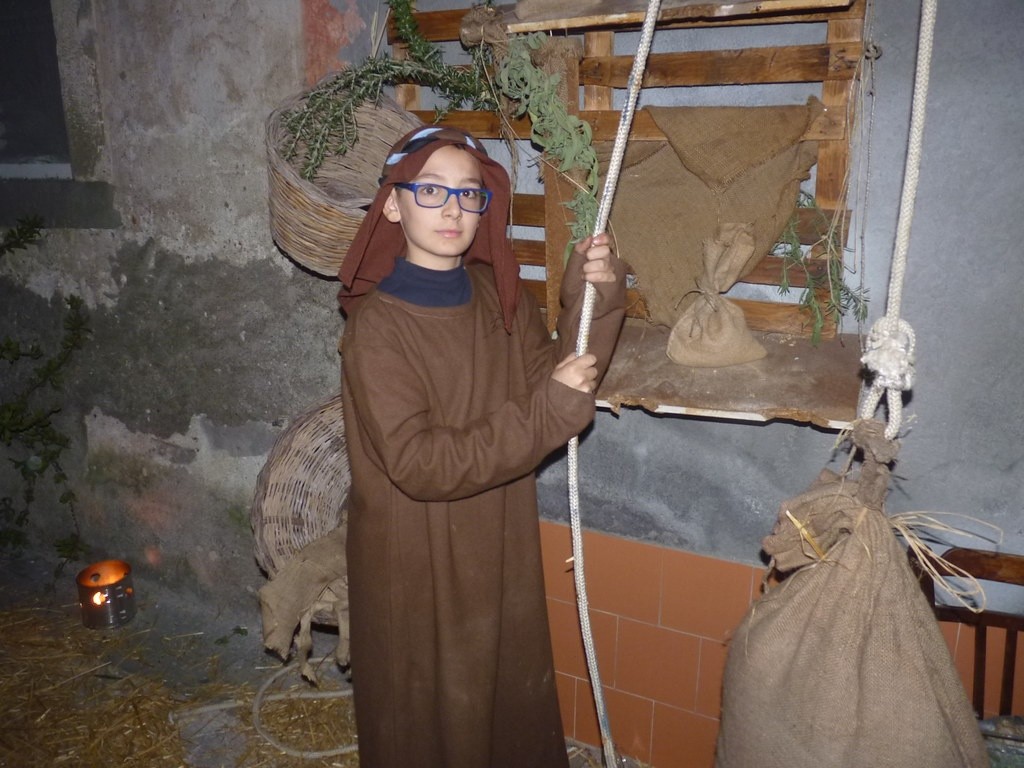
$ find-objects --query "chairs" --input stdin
[908,542,1024,723]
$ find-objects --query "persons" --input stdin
[340,125,626,768]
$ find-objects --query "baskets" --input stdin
[249,391,350,626]
[264,71,426,277]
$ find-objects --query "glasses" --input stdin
[393,182,493,213]
